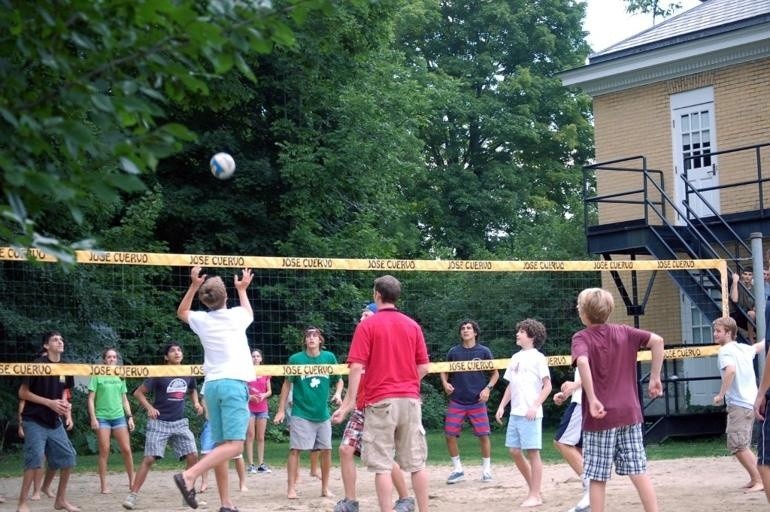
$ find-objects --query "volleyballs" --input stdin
[210,152,236,179]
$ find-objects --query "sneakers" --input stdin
[392,497,415,511]
[334,497,359,511]
[248,463,257,474]
[482,472,491,481]
[258,464,271,472]
[447,472,464,484]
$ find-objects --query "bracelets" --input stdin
[127,415,133,419]
[486,384,493,391]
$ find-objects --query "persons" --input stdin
[122,343,209,509]
[271,326,344,499]
[328,274,431,511]
[87,348,137,494]
[170,264,256,511]
[246,349,274,474]
[569,288,665,511]
[14,332,84,512]
[439,318,500,485]
[495,317,553,508]
[286,383,323,482]
[198,380,250,494]
[711,261,769,509]
[333,303,416,510]
[17,350,74,500]
[553,367,592,511]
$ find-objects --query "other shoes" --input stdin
[220,507,238,512]
[569,491,590,511]
[173,474,198,509]
[123,492,137,510]
